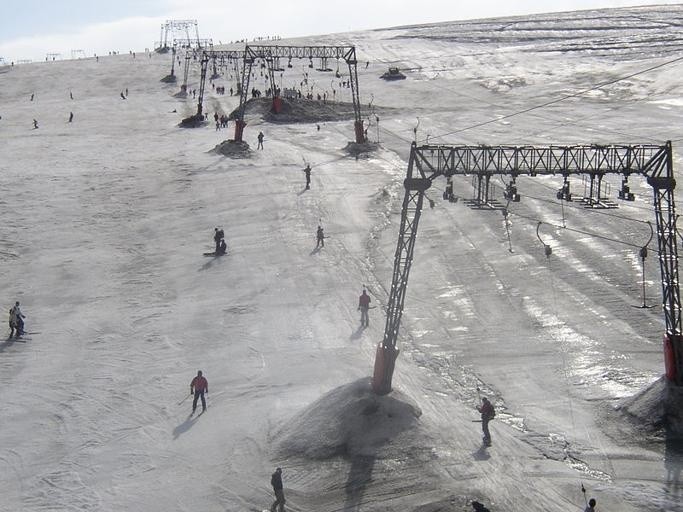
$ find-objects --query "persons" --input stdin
[358,290,371,327]
[271,467,286,511]
[211,65,351,101]
[214,228,222,254]
[317,226,324,247]
[31,92,74,128]
[585,499,596,512]
[257,132,264,150]
[214,112,228,131]
[476,397,493,445]
[46,51,135,62]
[473,502,488,511]
[177,55,197,98]
[9,301,26,339]
[190,371,208,413]
[121,88,128,99]
[304,165,311,186]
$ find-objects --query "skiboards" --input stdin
[1,330,42,343]
[190,404,210,418]
